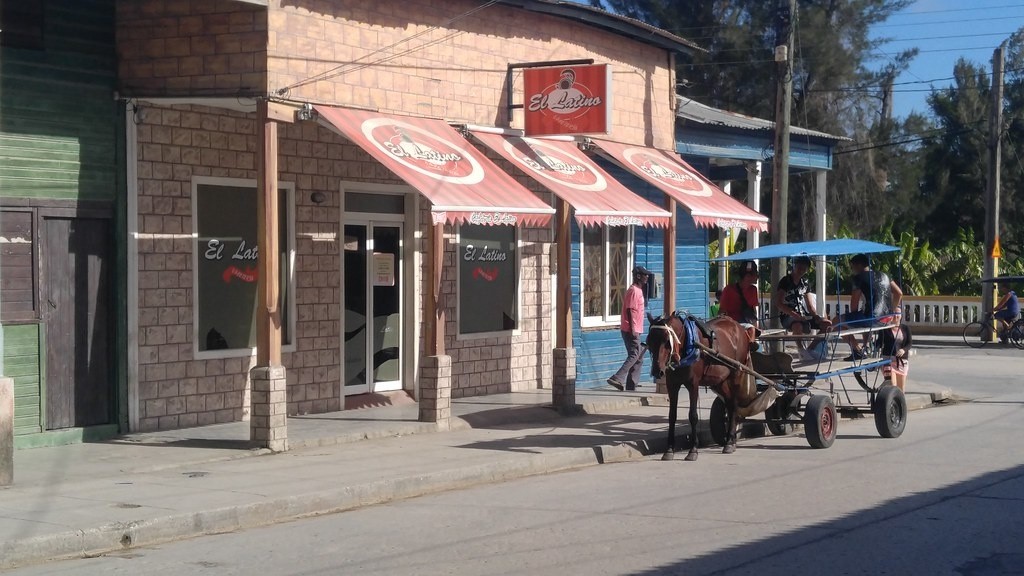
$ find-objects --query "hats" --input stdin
[632,266,651,274]
[735,261,758,275]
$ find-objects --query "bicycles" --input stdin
[962,276,1024,350]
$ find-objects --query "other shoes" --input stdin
[798,347,814,361]
[999,341,1008,344]
[607,379,624,391]
[809,349,825,361]
[843,349,872,361]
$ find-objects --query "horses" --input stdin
[645,310,749,461]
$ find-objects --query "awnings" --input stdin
[311,105,770,234]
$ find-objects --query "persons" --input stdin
[709,290,722,318]
[607,266,649,391]
[832,254,903,361]
[775,256,832,361]
[873,305,912,393]
[992,282,1019,336]
[717,261,760,351]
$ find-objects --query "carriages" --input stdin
[640,239,908,462]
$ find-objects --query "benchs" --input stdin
[753,322,896,343]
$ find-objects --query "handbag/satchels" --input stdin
[742,307,761,337]
